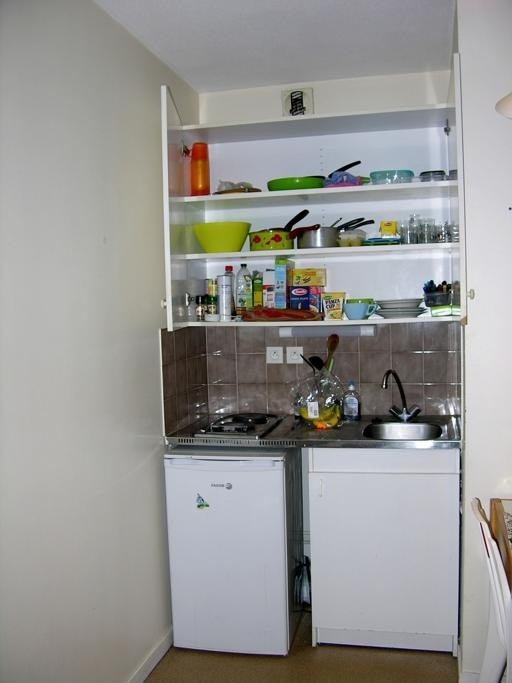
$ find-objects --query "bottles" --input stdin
[206,279,219,315]
[190,141,211,196]
[223,265,234,277]
[235,262,254,311]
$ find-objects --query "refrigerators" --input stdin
[162,447,304,657]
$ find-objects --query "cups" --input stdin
[345,302,377,318]
[402,213,458,243]
[350,298,376,302]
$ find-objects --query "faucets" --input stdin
[381,370,421,422]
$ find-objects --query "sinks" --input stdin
[360,413,461,441]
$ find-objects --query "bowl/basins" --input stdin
[371,170,414,184]
[191,221,248,252]
[420,171,446,182]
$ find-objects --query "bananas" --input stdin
[297,400,341,428]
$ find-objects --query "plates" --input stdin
[375,298,425,320]
[364,238,399,246]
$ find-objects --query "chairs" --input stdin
[471,497,512,683]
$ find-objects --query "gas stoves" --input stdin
[191,406,285,440]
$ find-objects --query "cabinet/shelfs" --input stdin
[307,448,460,659]
[160,53,467,333]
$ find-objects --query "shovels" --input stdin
[327,334,341,369]
[307,355,324,377]
[301,354,317,381]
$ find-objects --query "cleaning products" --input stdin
[342,378,362,423]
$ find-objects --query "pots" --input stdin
[296,213,375,250]
[249,224,320,250]
[248,209,309,232]
[268,160,366,190]
